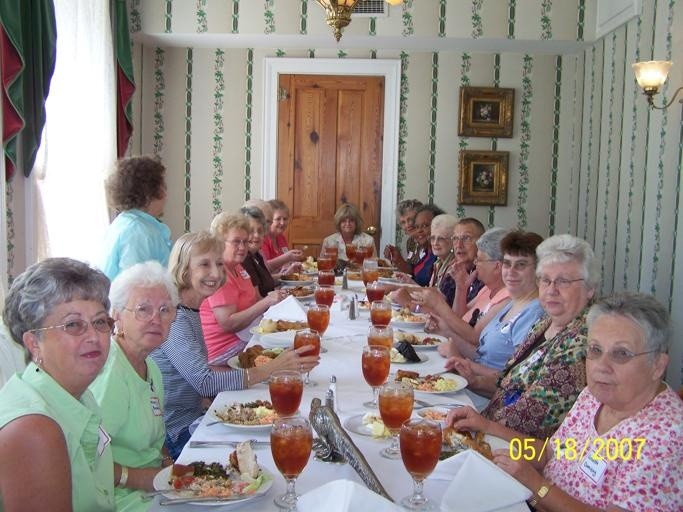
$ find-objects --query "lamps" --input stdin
[312,0,407,43]
[631,60,683,110]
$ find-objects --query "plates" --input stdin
[394,365,478,436]
[145,322,325,508]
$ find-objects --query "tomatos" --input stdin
[182,477,195,485]
[172,479,182,490]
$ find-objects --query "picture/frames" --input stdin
[458,149,507,206]
[458,85,515,138]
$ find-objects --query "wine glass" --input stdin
[360,344,391,410]
[366,324,394,355]
[397,416,441,512]
[292,327,322,391]
[268,414,316,509]
[265,370,307,418]
[263,238,443,333]
[378,379,414,463]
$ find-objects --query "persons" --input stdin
[0,199,320,512]
[100,155,174,280]
[320,203,377,266]
[384,199,682,512]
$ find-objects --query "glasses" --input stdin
[25,316,120,337]
[580,344,661,364]
[449,233,475,246]
[500,258,537,272]
[472,257,499,266]
[120,304,178,323]
[534,275,586,289]
[224,236,253,250]
